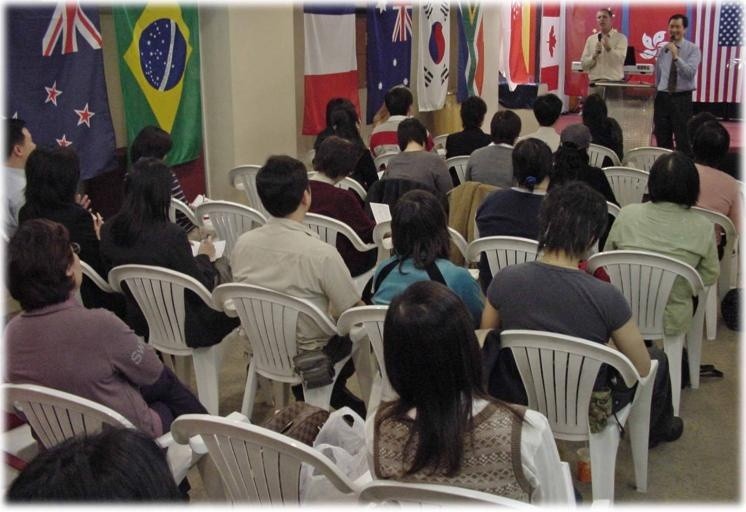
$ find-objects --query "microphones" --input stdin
[665,35,675,53]
[597,33,602,53]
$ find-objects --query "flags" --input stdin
[691,0,746,103]
[1,4,119,182]
[300,1,362,135]
[114,4,205,174]
[510,0,538,84]
[563,0,623,96]
[451,0,486,103]
[539,0,561,90]
[627,0,688,96]
[418,0,451,112]
[366,1,413,125]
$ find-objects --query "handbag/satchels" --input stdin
[256,401,332,447]
[588,385,614,435]
[294,351,336,391]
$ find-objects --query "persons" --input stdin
[4,86,743,508]
[652,13,701,158]
[579,7,628,121]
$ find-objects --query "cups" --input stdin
[575,448,593,484]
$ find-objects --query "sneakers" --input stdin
[648,414,683,448]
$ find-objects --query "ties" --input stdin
[668,49,678,97]
